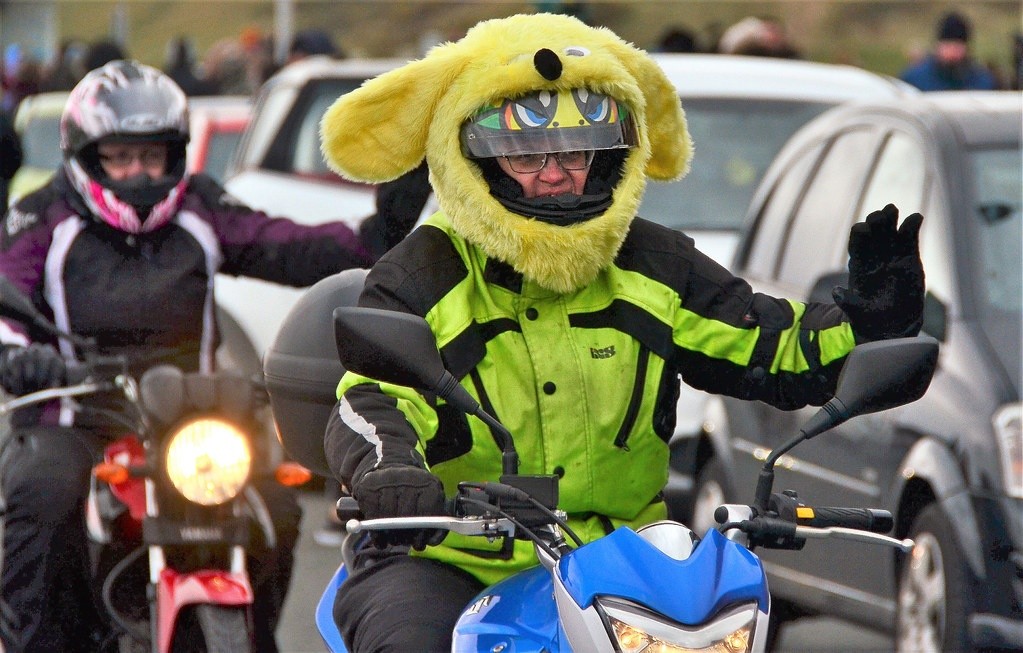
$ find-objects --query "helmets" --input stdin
[59,60,191,235]
[458,83,639,225]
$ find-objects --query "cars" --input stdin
[695,91,1023,653]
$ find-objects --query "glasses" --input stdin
[94,147,164,168]
[495,148,595,174]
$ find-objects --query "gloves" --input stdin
[360,158,432,260]
[0,344,64,396]
[352,466,451,552]
[831,203,925,340]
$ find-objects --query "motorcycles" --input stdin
[0,275,315,653]
[267,267,939,653]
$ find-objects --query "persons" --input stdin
[653,13,803,59]
[1,18,348,223]
[320,12,926,653]
[0,59,433,653]
[905,9,992,93]
